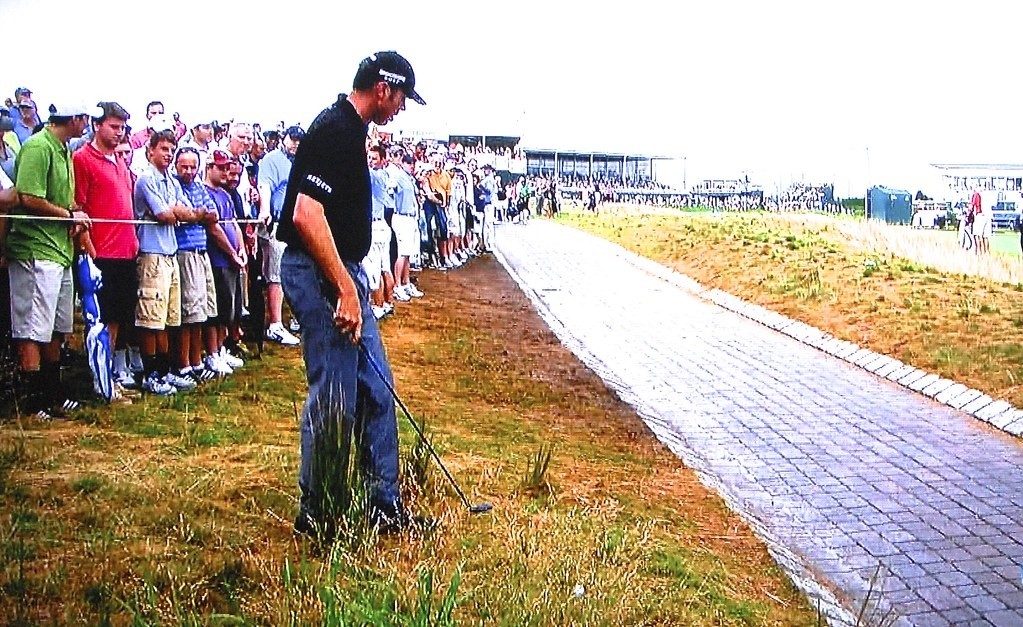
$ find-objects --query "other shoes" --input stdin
[368,501,435,536]
[292,495,357,543]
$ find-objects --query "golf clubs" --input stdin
[317,270,494,513]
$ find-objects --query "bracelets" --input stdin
[67,208,74,218]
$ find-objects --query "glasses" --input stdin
[394,153,402,157]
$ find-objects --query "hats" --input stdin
[19,99,36,110]
[148,115,174,132]
[282,126,305,148]
[401,155,417,164]
[15,87,33,99]
[205,147,237,167]
[388,145,403,154]
[366,51,426,106]
[190,113,213,131]
[49,92,105,119]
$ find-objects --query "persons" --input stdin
[276,50,434,538]
[0,86,851,423]
[966,188,992,253]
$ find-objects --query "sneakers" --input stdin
[23,236,490,426]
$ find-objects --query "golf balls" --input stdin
[573,586,586,596]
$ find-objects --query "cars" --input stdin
[993,201,1021,231]
